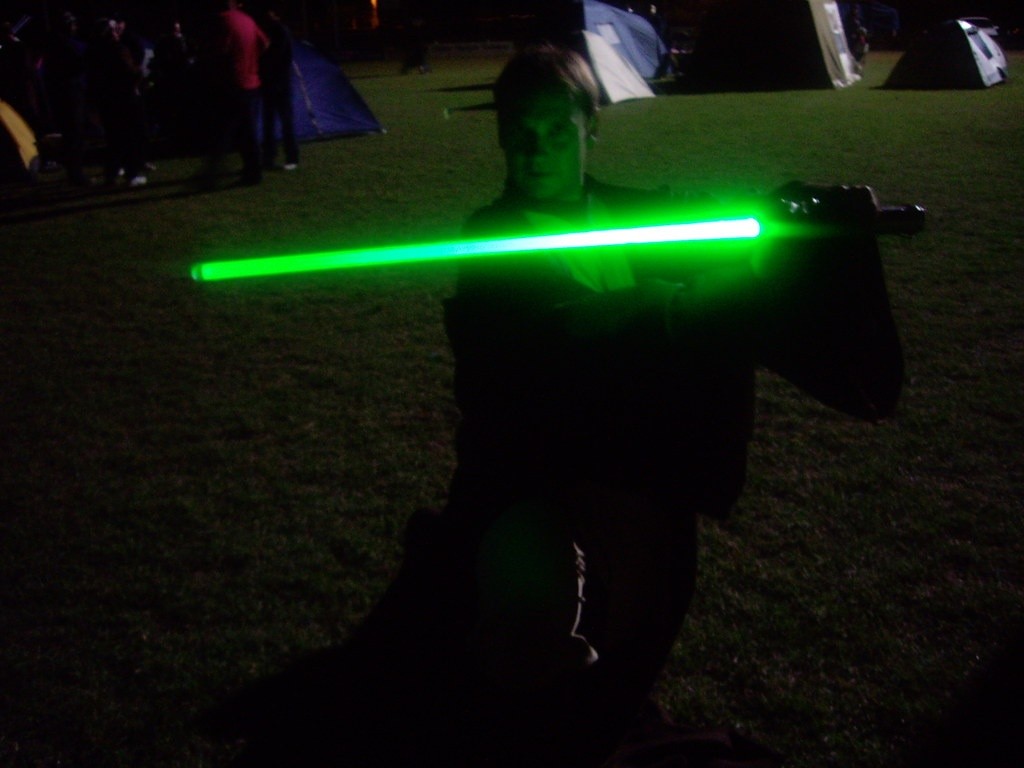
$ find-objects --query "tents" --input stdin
[197,25,389,145]
[542,25,658,105]
[836,0,900,59]
[516,0,668,81]
[686,0,862,92]
[885,20,1007,90]
[961,16,1005,52]
[0,102,41,171]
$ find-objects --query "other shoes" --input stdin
[284,163,296,171]
[119,161,158,187]
[230,166,263,187]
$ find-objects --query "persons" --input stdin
[0,0,867,199]
[202,42,903,753]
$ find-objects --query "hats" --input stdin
[62,11,76,23]
